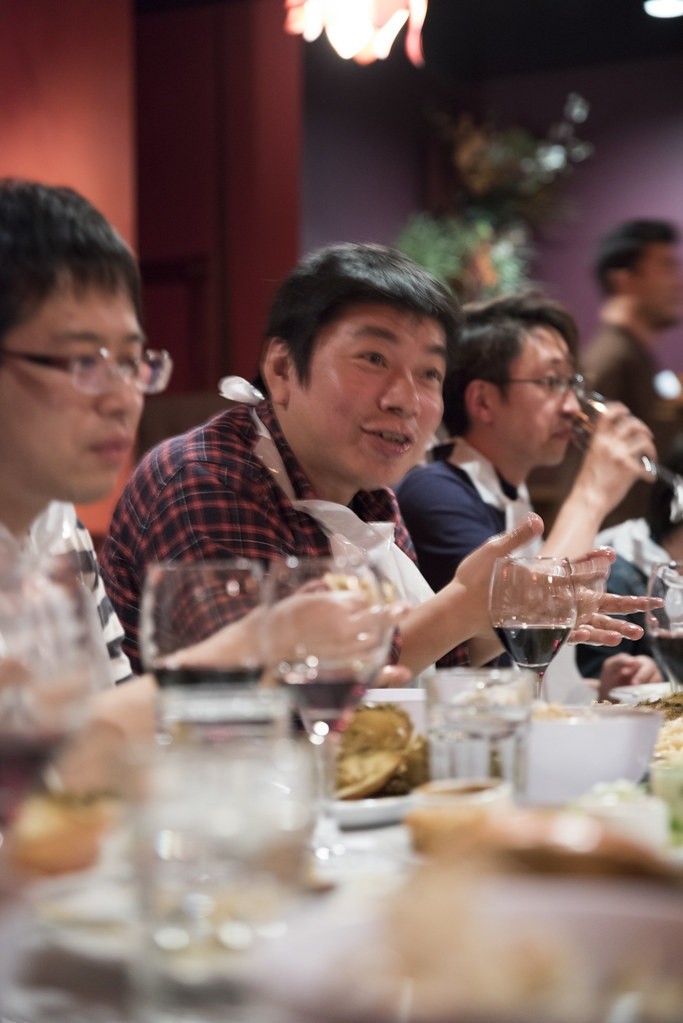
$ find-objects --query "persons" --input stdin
[98,241,665,693]
[578,216,683,420]
[0,178,410,686]
[396,287,658,701]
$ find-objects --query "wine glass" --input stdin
[568,394,683,524]
[250,559,395,867]
[486,554,579,711]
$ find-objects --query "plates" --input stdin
[608,680,672,711]
[327,788,428,831]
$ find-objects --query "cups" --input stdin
[1,525,150,819]
[425,672,538,803]
[645,562,683,697]
[137,560,269,712]
